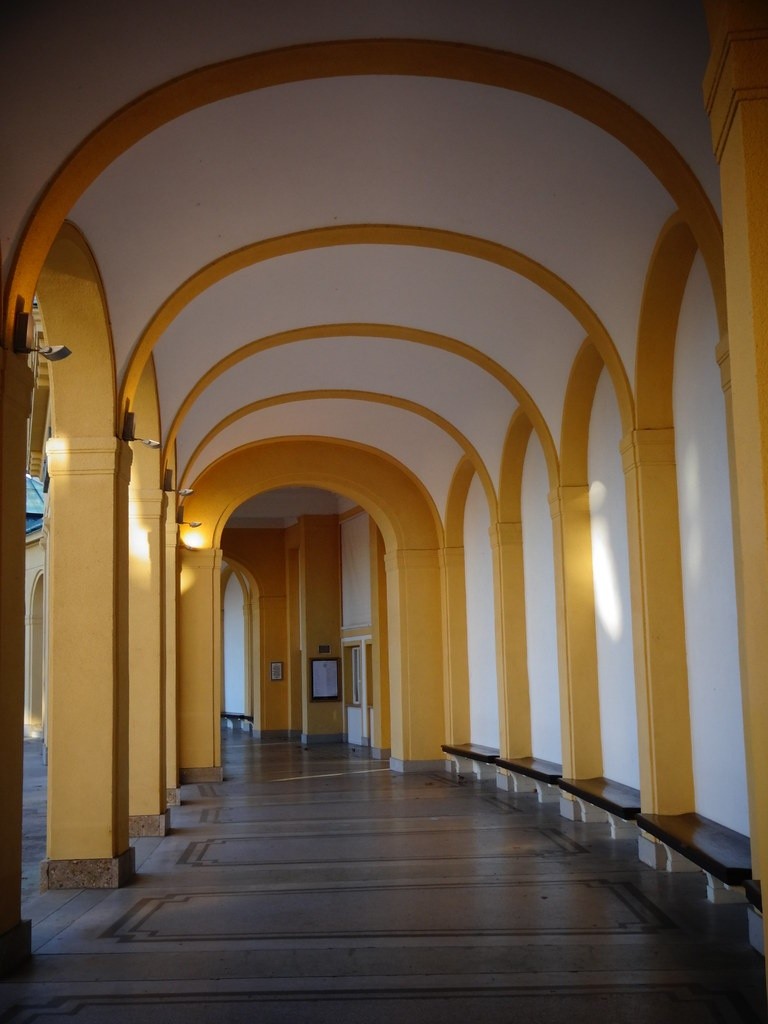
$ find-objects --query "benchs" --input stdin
[555,777,640,840]
[744,881,762,913]
[221,712,253,739]
[636,812,752,906]
[495,757,562,804]
[440,743,500,781]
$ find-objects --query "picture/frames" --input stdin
[309,657,342,703]
[271,662,283,681]
